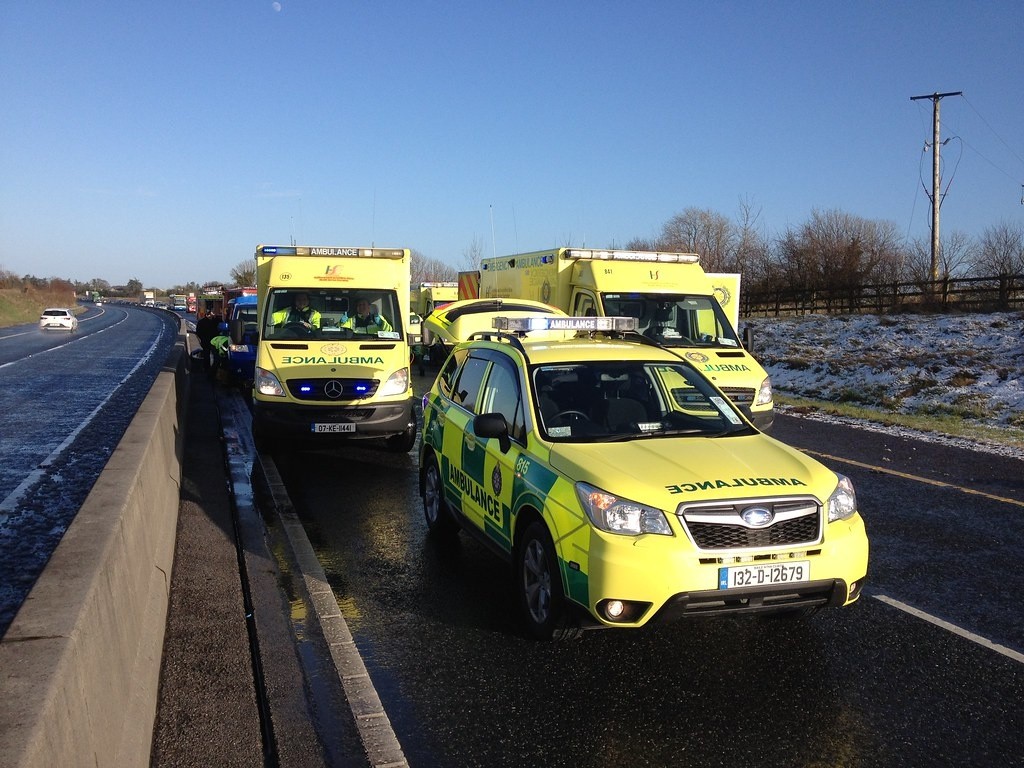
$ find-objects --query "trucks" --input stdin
[247,246,417,453]
[224,287,258,318]
[457,248,774,439]
[411,282,459,317]
[226,296,259,375]
[89,291,100,302]
[195,294,225,325]
[186,297,196,314]
[139,291,155,305]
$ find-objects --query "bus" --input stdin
[169,296,186,311]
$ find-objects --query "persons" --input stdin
[195,308,225,378]
[271,291,321,333]
[335,295,392,334]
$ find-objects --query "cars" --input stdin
[78,296,167,311]
[39,308,79,334]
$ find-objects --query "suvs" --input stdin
[416,297,870,643]
